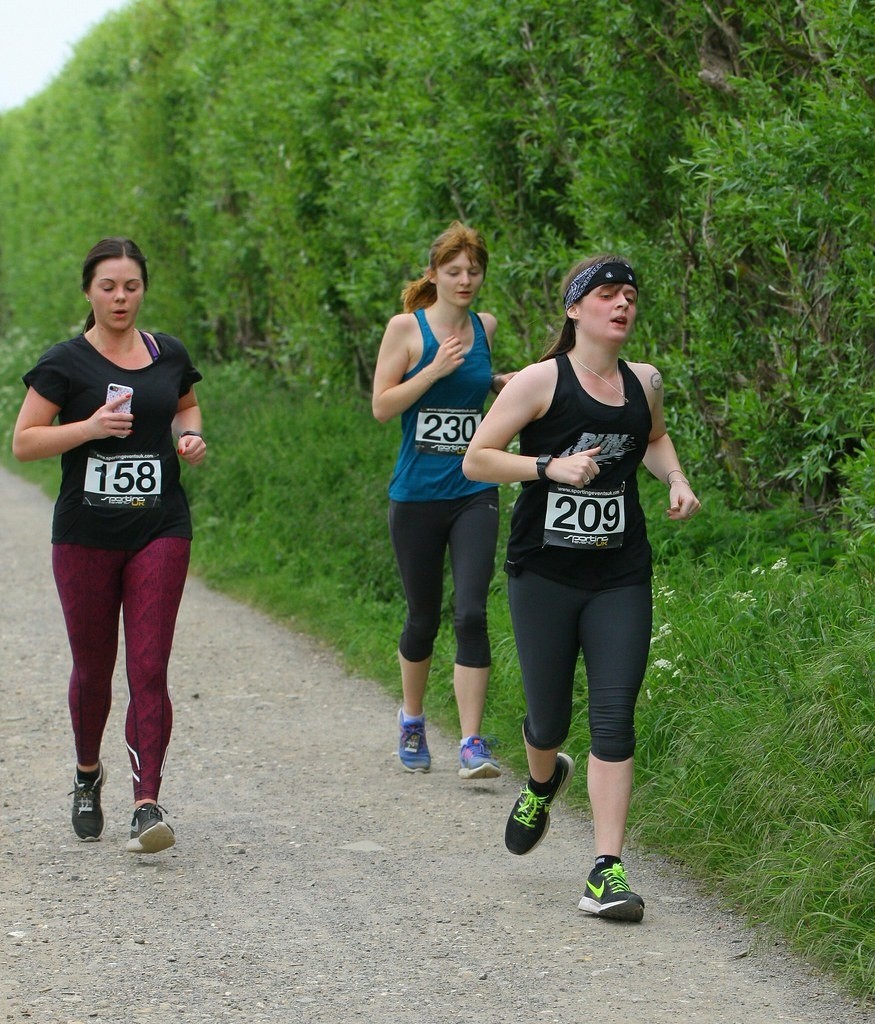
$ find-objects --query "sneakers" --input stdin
[68,761,106,842]
[577,863,645,922]
[504,752,575,856]
[458,736,502,779]
[398,708,431,773]
[126,803,176,854]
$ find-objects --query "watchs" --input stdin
[536,454,553,480]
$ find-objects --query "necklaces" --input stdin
[571,350,629,403]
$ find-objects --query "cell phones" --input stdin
[106,383,133,438]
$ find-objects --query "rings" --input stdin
[584,476,591,485]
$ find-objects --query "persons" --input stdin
[370,222,518,778]
[462,255,702,922]
[12,240,206,853]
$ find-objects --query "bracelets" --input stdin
[178,431,204,440]
[422,368,433,384]
[492,374,500,395]
[667,469,690,486]
